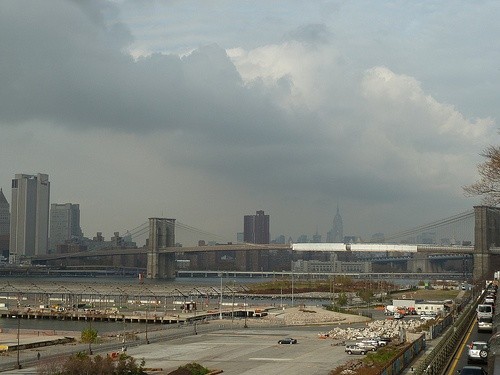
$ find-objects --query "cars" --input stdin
[394,312,403,318]
[278,338,297,344]
[456,365,487,375]
[484,285,498,306]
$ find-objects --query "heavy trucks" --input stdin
[384,305,416,316]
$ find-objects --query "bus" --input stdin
[420,314,436,320]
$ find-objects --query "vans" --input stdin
[476,303,494,320]
[477,315,493,334]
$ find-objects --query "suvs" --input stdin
[345,338,387,355]
[466,340,488,364]
